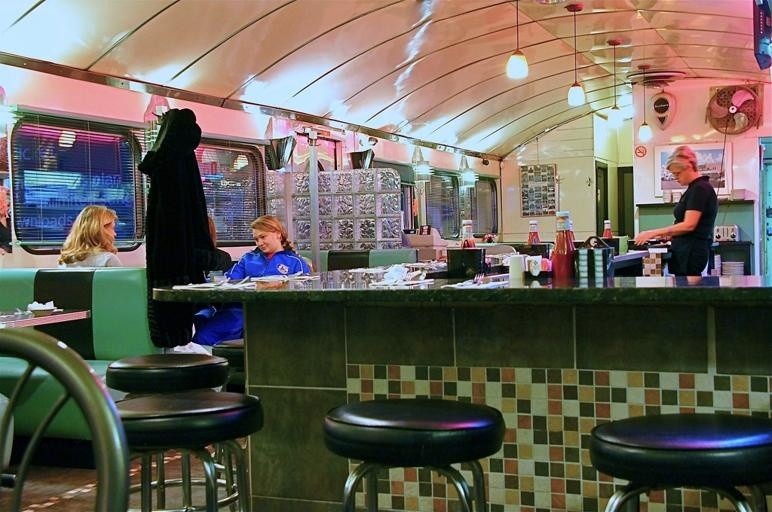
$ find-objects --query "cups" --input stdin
[210,270,222,282]
[711,254,721,276]
[213,275,227,284]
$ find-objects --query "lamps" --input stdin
[455,155,480,191]
[606,37,624,130]
[563,3,587,107]
[505,1,529,80]
[637,63,655,142]
[410,146,435,183]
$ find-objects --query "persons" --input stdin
[57,204,122,267]
[200,215,232,276]
[193,214,311,345]
[635,146,719,276]
[0,186,12,255]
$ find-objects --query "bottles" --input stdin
[528,220,540,245]
[603,220,612,239]
[509,255,525,288]
[552,211,575,288]
[461,220,476,248]
[569,221,575,240]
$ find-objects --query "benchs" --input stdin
[0,267,164,467]
[299,249,416,271]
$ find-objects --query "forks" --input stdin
[459,272,484,287]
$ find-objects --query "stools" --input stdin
[113,392,264,512]
[322,399,506,512]
[588,413,772,512]
[106,355,230,390]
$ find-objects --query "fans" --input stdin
[706,85,762,136]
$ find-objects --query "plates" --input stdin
[722,262,745,275]
[31,309,54,316]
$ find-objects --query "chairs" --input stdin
[0,329,131,512]
